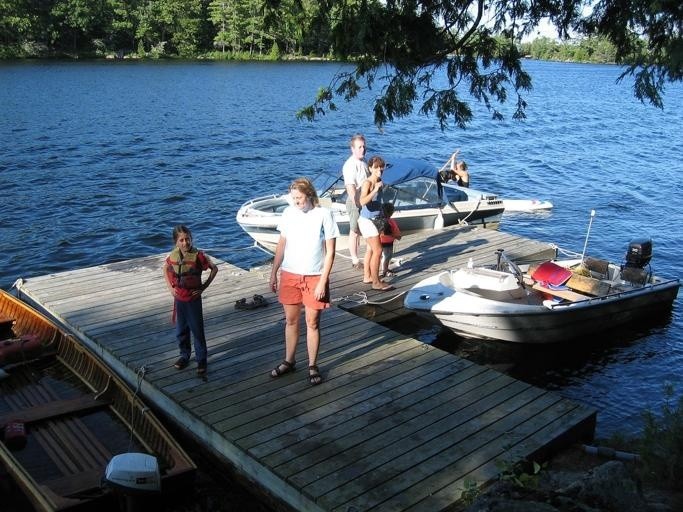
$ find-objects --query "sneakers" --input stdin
[352,261,363,272]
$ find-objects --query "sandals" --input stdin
[253,293,268,308]
[233,298,255,309]
[379,270,397,278]
[308,366,321,385]
[270,360,295,378]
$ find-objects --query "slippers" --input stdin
[372,284,395,291]
[196,363,206,373]
[174,359,189,369]
[363,281,384,284]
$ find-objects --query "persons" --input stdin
[379,203,401,277]
[451,154,469,188]
[268,178,341,386]
[164,226,218,373]
[437,170,457,196]
[342,135,371,271]
[357,155,396,292]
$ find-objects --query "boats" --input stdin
[0,287,201,511]
[232,177,505,261]
[402,210,681,341]
